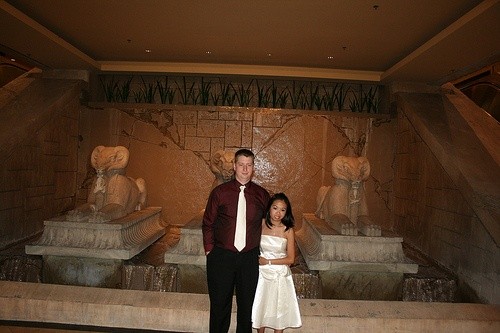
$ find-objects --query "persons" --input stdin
[202,148,271,333]
[251,192,302,333]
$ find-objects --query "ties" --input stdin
[234,186,246,252]
